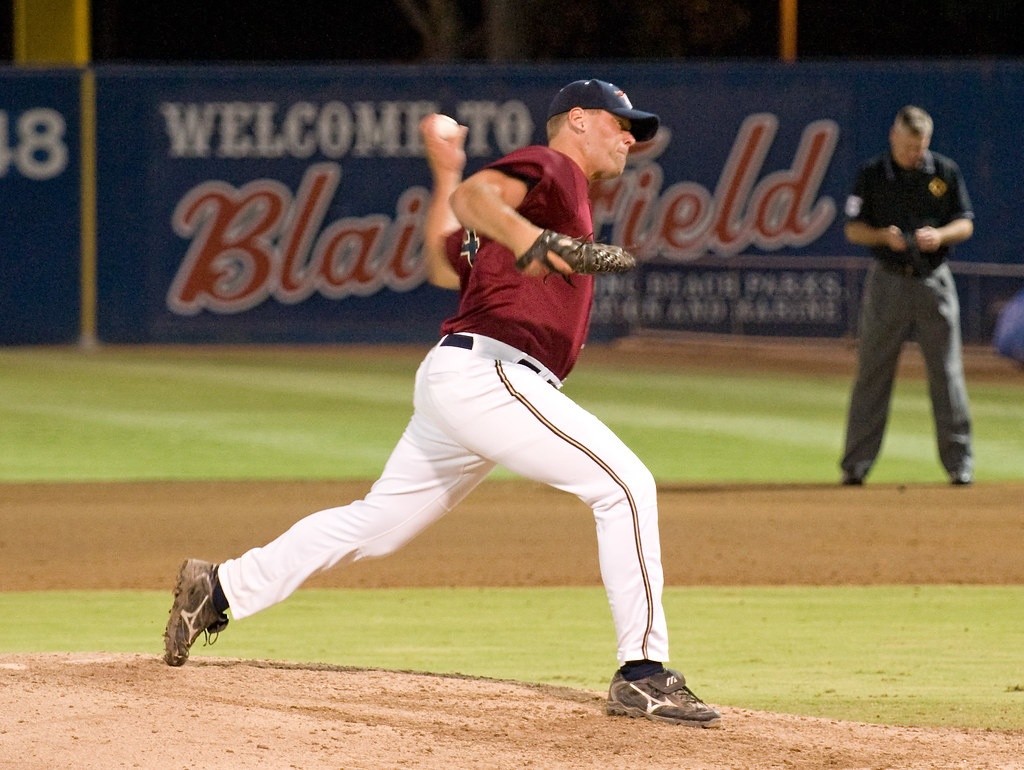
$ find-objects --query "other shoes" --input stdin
[843,474,862,485]
[952,472,971,484]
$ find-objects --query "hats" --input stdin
[545,79,660,142]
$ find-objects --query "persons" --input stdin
[840,108,976,486]
[163,80,722,734]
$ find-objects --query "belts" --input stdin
[440,332,563,390]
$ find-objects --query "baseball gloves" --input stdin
[511,226,640,282]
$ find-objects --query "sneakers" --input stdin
[606,668,722,727]
[162,558,229,667]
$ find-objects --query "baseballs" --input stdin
[431,111,463,143]
